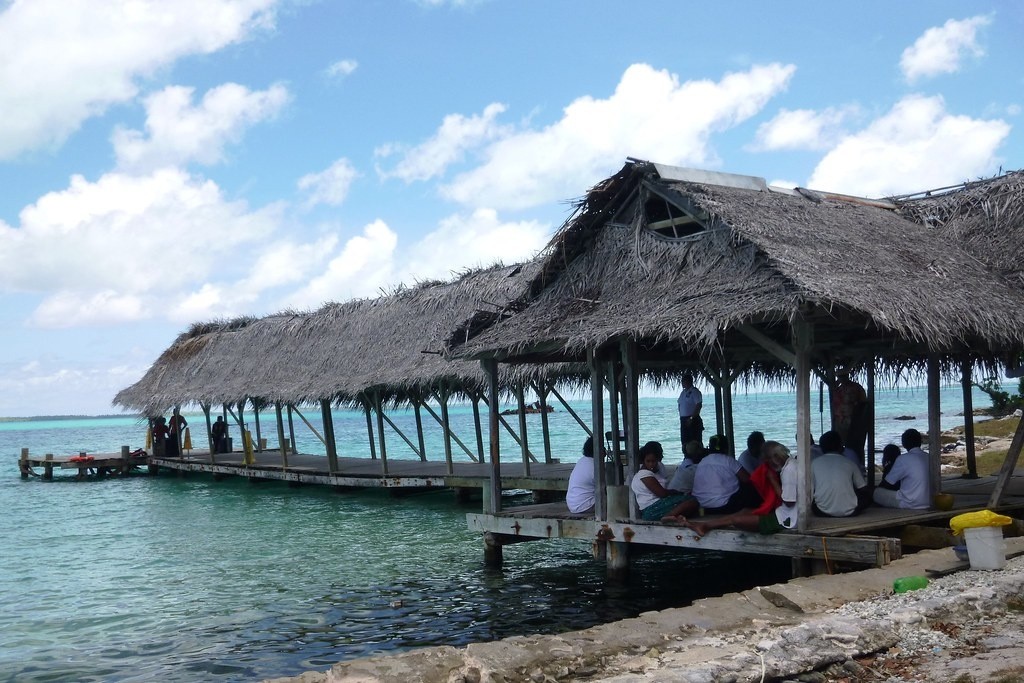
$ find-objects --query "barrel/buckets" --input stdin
[963,526,1008,569]
[936,494,955,510]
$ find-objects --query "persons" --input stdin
[168,409,188,456]
[211,416,227,454]
[690,435,765,516]
[832,371,868,468]
[566,437,607,513]
[153,418,168,456]
[737,431,765,475]
[666,440,703,495]
[872,428,931,511]
[676,375,704,462]
[810,430,867,519]
[624,441,699,527]
[678,432,860,537]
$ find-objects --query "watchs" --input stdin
[690,416,693,419]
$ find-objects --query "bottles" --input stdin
[893,575,931,593]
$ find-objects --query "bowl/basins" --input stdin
[953,546,968,560]
